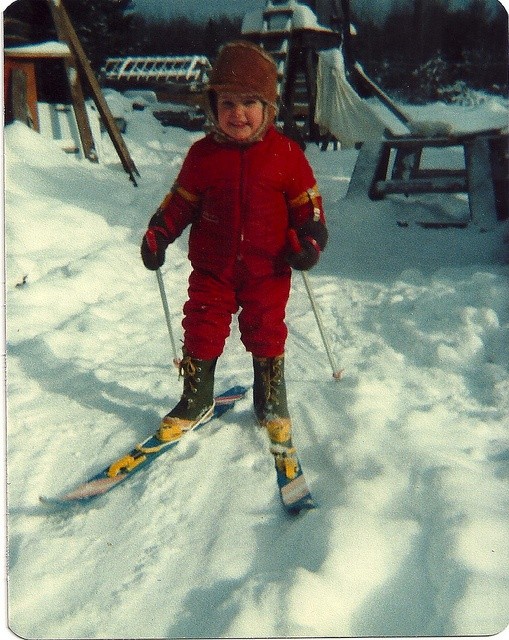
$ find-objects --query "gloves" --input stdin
[284,221,328,270]
[140,230,168,268]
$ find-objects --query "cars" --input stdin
[153,109,207,127]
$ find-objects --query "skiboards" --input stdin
[36,385,315,516]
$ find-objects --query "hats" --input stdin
[205,40,278,146]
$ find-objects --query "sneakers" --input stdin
[253,355,292,445]
[158,356,219,441]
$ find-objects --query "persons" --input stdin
[141,43,328,444]
